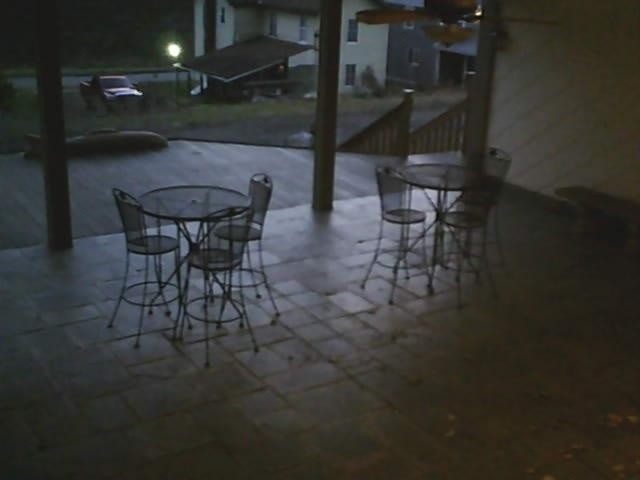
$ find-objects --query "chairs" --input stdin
[361,165,433,305]
[173,204,256,366]
[204,173,280,316]
[427,173,503,309]
[446,147,513,265]
[108,188,192,349]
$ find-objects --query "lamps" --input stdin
[423,19,475,48]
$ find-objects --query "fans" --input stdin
[354,0,564,47]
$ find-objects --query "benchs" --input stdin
[554,186,640,251]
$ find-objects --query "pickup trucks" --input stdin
[79,74,144,111]
